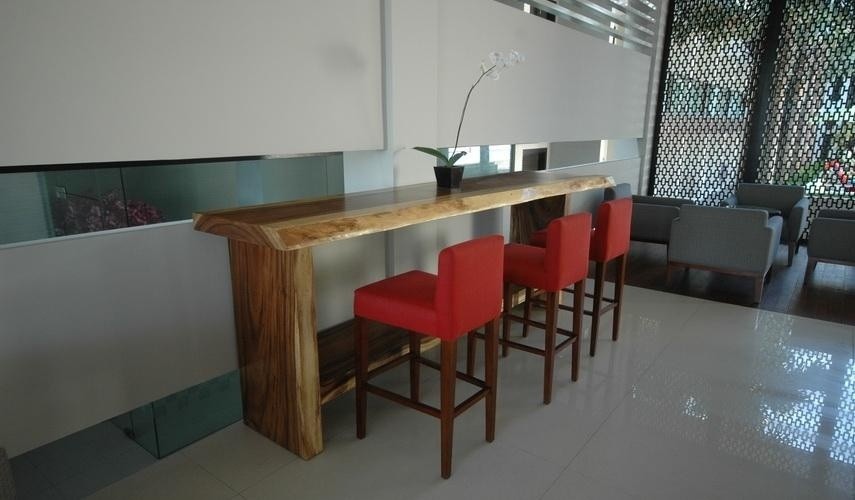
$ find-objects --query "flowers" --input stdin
[408,45,527,167]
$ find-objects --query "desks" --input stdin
[176,170,616,461]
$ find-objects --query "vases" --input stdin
[434,165,463,189]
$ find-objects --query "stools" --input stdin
[348,234,505,479]
[466,211,594,404]
[522,197,636,357]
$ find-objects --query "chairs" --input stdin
[603,182,855,304]
